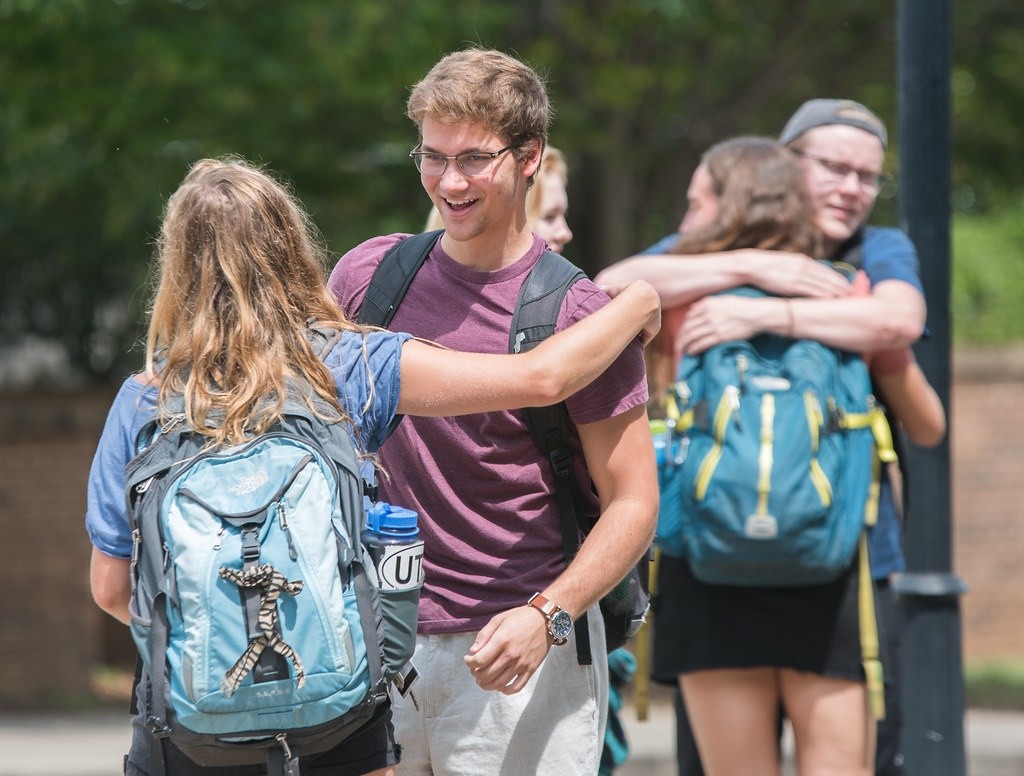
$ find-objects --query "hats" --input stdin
[778,97,889,152]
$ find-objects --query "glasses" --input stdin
[791,144,885,198]
[410,142,516,177]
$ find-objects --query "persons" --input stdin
[324,48,660,774]
[85,158,662,774]
[591,96,944,775]
[423,145,574,256]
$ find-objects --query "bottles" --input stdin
[645,416,676,471]
[360,503,426,596]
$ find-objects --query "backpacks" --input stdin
[648,284,881,583]
[120,324,429,770]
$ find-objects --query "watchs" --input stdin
[527,592,574,646]
